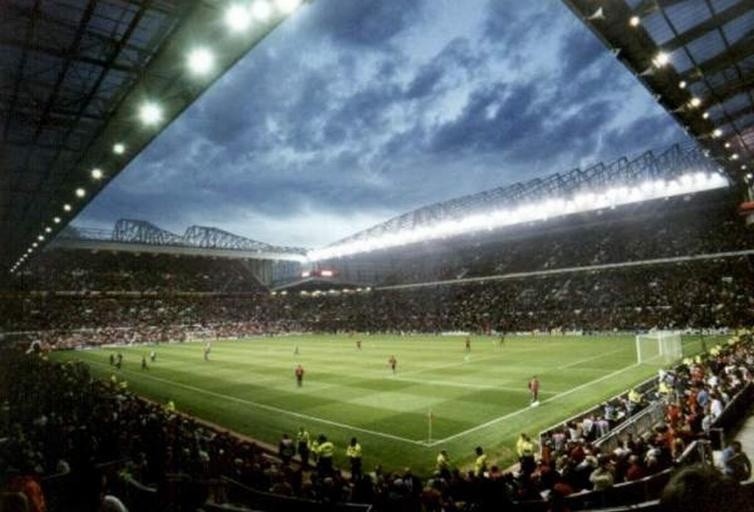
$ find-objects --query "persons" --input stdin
[114,387,753,508]
[293,363,305,387]
[148,349,156,363]
[651,326,752,386]
[526,374,541,407]
[0,340,114,511]
[0,246,272,350]
[272,290,369,338]
[387,354,397,375]
[495,333,505,354]
[139,354,149,375]
[462,335,472,361]
[369,204,751,334]
[202,341,211,361]
[291,343,300,356]
[115,353,122,370]
[355,337,362,351]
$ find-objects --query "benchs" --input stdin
[513,440,754,511]
[101,472,373,512]
[695,381,754,450]
